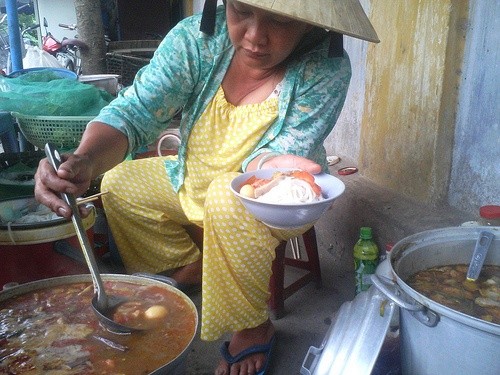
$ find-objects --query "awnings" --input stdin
[0,0,35,14]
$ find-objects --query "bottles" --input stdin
[353,225,375,296]
[375,242,400,338]
[476,205,500,227]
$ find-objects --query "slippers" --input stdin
[221,333,276,375]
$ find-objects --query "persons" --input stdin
[33,0,381,375]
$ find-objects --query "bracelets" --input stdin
[258,152,280,170]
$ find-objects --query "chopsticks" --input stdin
[75,191,110,207]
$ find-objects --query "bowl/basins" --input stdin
[0,167,39,202]
[77,72,119,95]
[228,169,346,230]
[0,197,93,230]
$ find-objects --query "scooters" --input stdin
[40,17,76,72]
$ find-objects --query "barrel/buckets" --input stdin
[0,208,96,293]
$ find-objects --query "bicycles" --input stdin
[0,4,39,90]
[58,23,111,75]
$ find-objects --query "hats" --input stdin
[238,0,381,44]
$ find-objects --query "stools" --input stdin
[184,223,322,322]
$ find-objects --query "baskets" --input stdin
[11,110,96,149]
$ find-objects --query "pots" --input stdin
[0,272,198,375]
[370,226,500,375]
